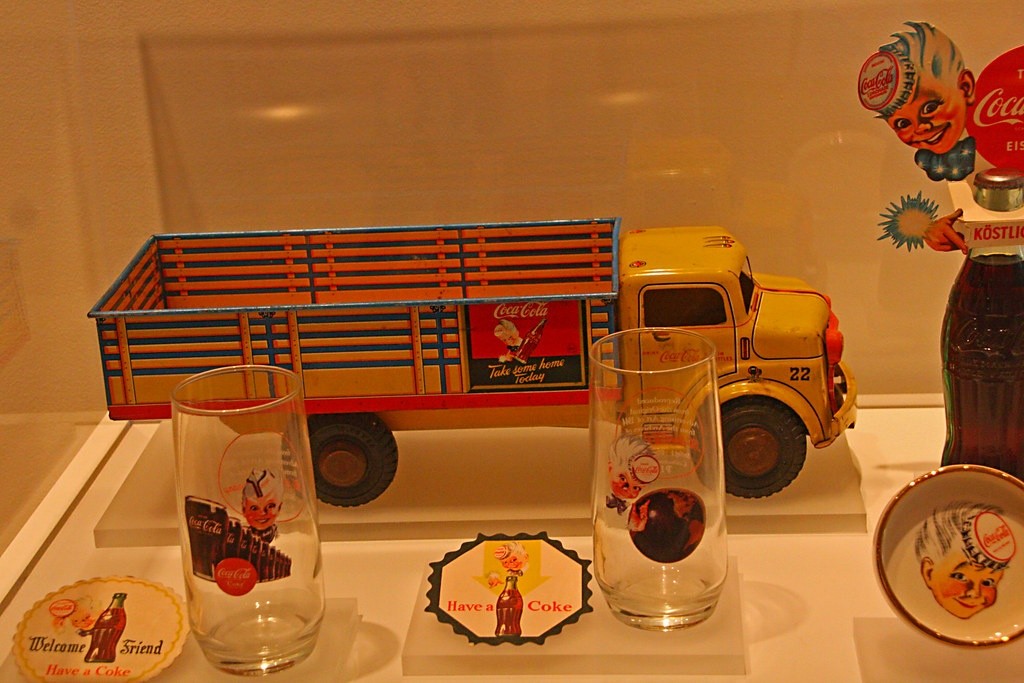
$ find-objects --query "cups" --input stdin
[589,326,729,632]
[167,365,327,678]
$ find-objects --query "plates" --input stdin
[870,464,1024,650]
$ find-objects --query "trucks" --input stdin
[90,215,864,503]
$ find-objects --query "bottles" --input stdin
[84,593,127,662]
[939,167,1024,482]
[495,576,523,636]
